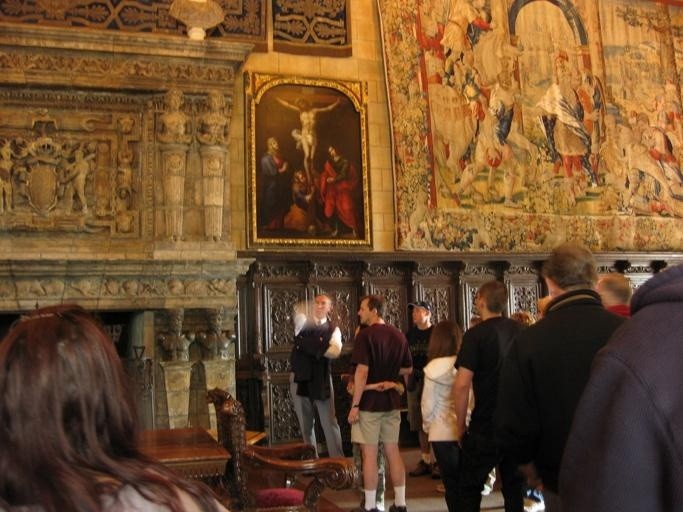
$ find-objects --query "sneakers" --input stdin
[409,459,432,477]
[432,462,441,479]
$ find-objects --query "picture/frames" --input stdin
[244,68,374,249]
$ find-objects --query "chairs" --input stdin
[207,386,358,512]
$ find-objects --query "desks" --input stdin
[135,426,231,481]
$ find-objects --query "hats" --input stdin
[407,301,430,311]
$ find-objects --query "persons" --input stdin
[421,320,475,511]
[0,302,233,512]
[345,295,413,511]
[288,293,344,458]
[404,301,441,479]
[452,244,683,512]
[261,92,359,239]
